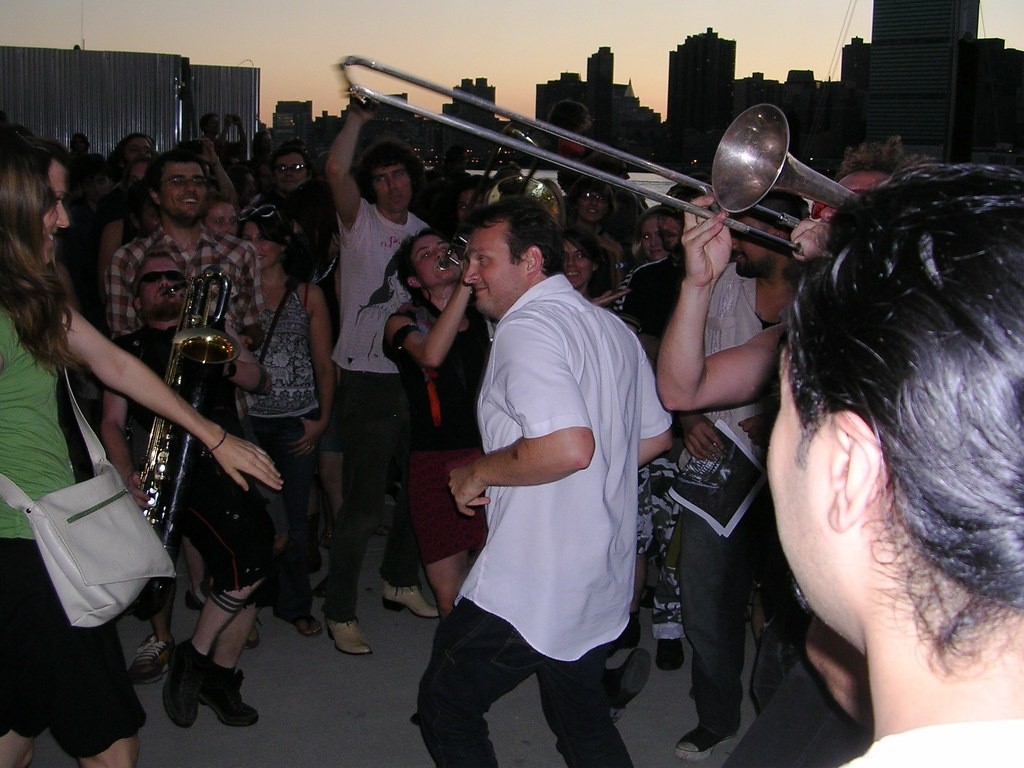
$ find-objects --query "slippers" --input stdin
[293,614,322,637]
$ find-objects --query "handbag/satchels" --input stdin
[22,462,178,622]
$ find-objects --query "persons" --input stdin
[0,99,1024,768]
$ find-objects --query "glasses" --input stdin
[154,176,206,188]
[237,205,285,226]
[276,163,306,175]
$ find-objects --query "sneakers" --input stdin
[186,580,212,609]
[199,670,259,726]
[129,632,175,684]
[245,625,260,648]
[674,724,740,761]
[605,648,650,724]
[164,640,205,727]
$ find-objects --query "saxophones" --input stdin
[125,265,244,620]
[432,245,497,343]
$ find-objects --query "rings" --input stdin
[306,444,312,449]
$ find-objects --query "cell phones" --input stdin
[180,140,204,155]
[226,115,237,121]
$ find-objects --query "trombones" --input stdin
[334,51,861,258]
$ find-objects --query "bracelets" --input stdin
[208,428,228,452]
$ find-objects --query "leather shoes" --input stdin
[326,619,372,655]
[382,582,439,619]
